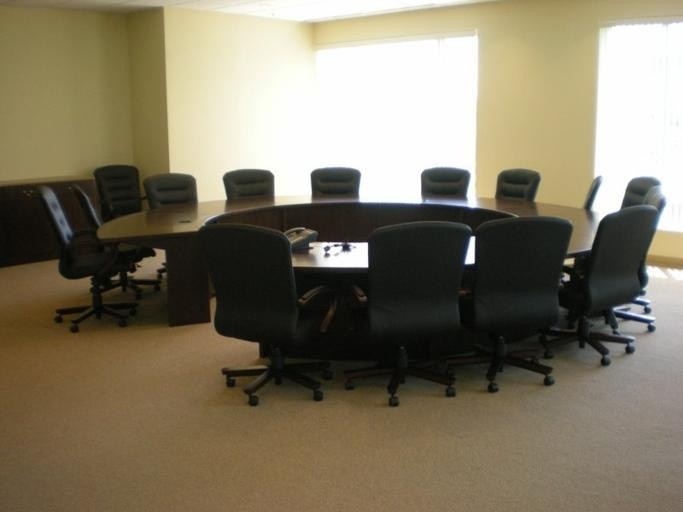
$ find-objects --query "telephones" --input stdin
[282,226,318,252]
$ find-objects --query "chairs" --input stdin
[70,185,160,297]
[460,218,574,393]
[584,176,601,209]
[224,169,273,198]
[493,168,540,199]
[550,206,655,365]
[94,166,162,221]
[616,177,667,316]
[144,172,196,276]
[422,167,468,196]
[37,184,139,332]
[310,168,360,196]
[349,222,472,406]
[207,223,352,406]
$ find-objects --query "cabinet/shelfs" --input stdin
[0,175,100,269]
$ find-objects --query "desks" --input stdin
[98,193,606,326]
